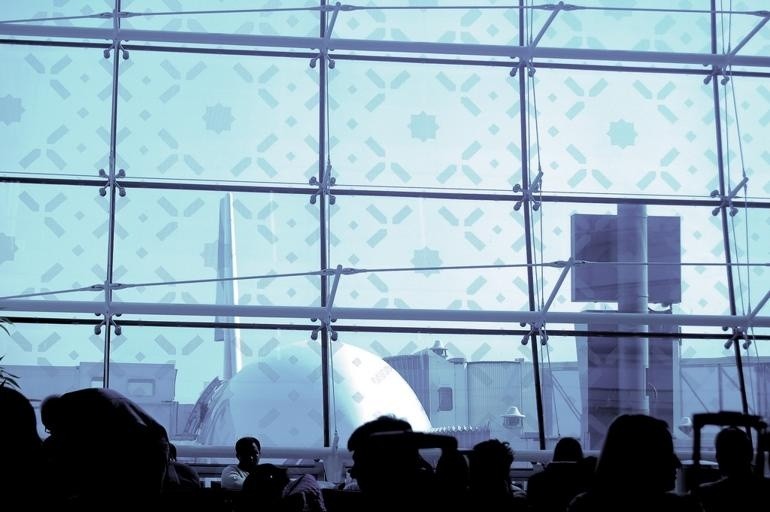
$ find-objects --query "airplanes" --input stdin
[158,191,443,469]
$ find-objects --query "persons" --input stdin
[0,389,769,511]
